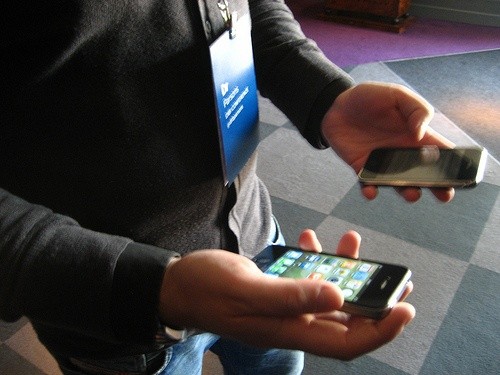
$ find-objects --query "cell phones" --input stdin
[252,245,412,319]
[357,147,489,188]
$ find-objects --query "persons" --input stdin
[0,0,466,375]
